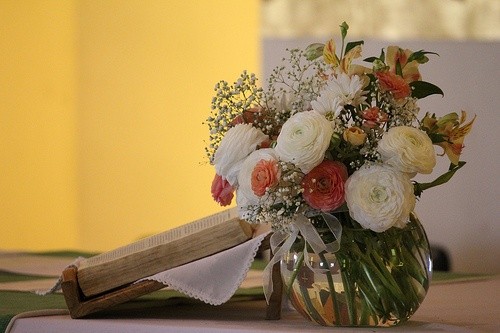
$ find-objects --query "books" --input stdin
[76,205,256,298]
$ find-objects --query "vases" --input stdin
[280,209,434,327]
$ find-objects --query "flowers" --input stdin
[198,21,477,326]
